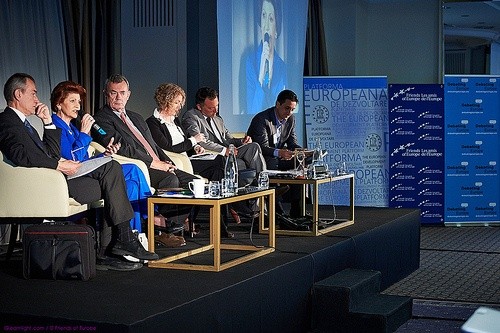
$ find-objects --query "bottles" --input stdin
[224,144,238,195]
[312,137,323,171]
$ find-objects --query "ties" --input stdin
[24,119,42,147]
[206,117,215,135]
[119,113,159,162]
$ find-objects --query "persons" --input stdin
[49,80,185,235]
[239,0,286,115]
[145,82,236,239]
[182,88,267,216]
[0,73,160,271]
[246,90,316,221]
[90,75,187,248]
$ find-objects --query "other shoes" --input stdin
[275,184,289,196]
[154,233,186,248]
[305,212,313,218]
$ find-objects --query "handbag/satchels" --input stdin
[23,222,96,281]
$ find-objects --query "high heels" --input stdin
[221,229,234,238]
[230,206,260,224]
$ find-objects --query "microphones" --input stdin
[78,110,106,135]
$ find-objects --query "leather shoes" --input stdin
[146,218,184,234]
[95,255,143,271]
[109,238,158,260]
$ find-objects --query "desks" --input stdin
[259,172,355,237]
[147,185,275,272]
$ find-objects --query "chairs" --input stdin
[0,132,246,261]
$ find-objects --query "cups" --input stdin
[258,172,269,190]
[221,179,234,197]
[338,161,346,174]
[188,179,205,198]
[208,181,220,198]
